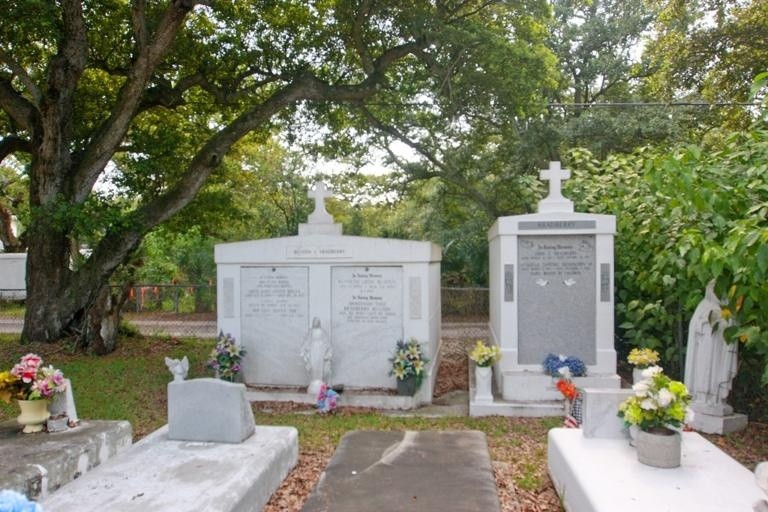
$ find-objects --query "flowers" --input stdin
[633,365,692,431]
[207,329,247,382]
[469,341,499,366]
[626,348,660,368]
[618,397,643,429]
[555,380,583,401]
[318,385,340,415]
[0,353,66,405]
[542,352,586,390]
[387,339,430,386]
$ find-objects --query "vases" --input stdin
[631,367,659,386]
[570,398,582,424]
[397,374,415,396]
[637,426,681,467]
[630,425,641,448]
[16,400,52,434]
